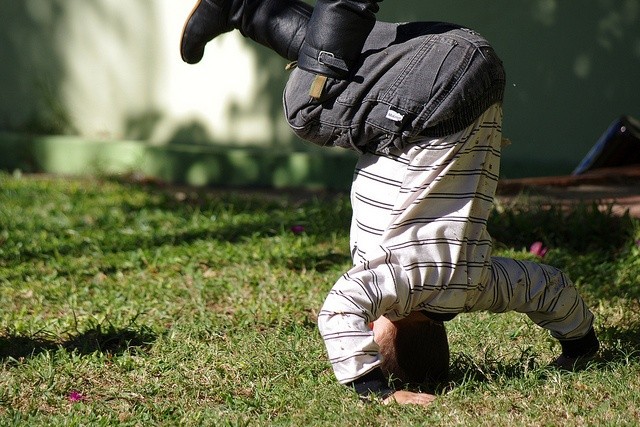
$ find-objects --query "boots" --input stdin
[179,0,316,66]
[297,0,379,81]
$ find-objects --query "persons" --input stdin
[181,0,599,405]
